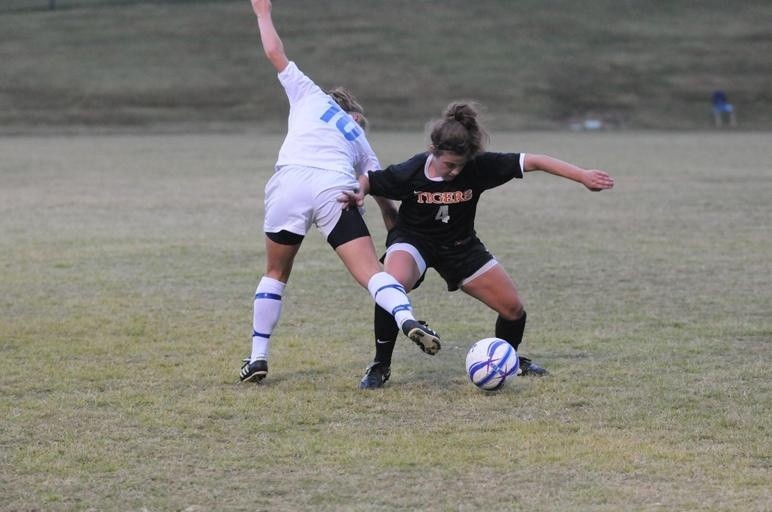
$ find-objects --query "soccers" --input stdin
[465,337,520,391]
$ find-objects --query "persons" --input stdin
[236,0,442,385]
[339,98,616,390]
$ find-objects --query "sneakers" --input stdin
[402,320,442,357]
[359,361,392,390]
[519,356,554,378]
[239,357,269,384]
